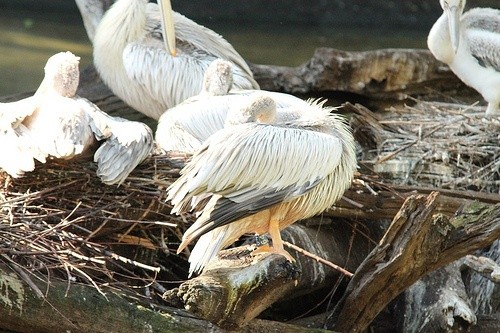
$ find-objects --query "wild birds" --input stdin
[426,0,500,116]
[155,57,358,287]
[73,0,261,120]
[0,50,153,194]
